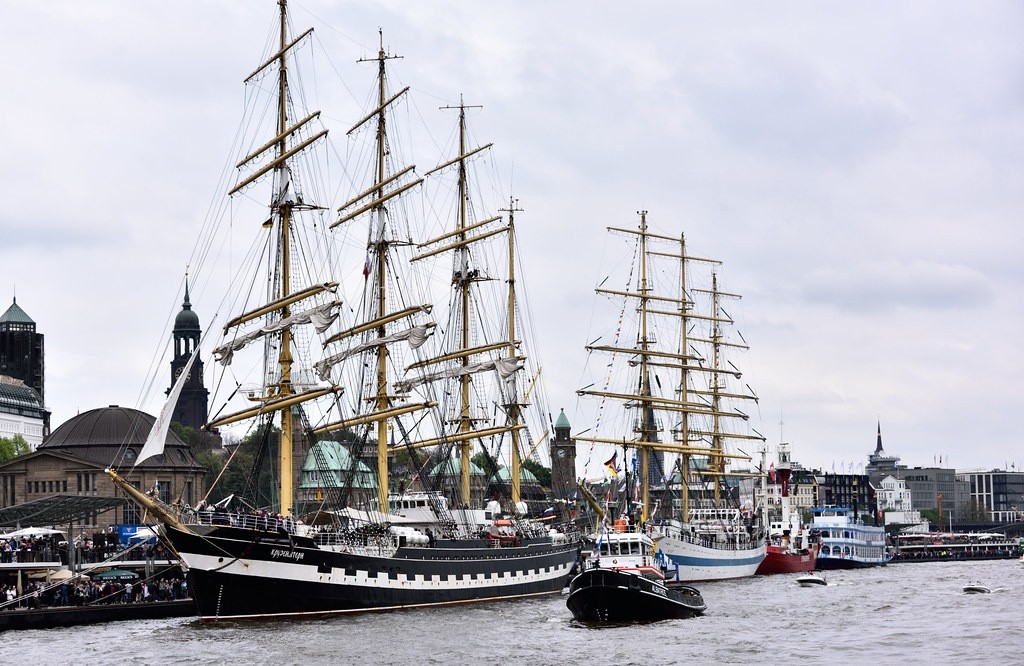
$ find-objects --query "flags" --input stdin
[769,463,776,483]
[604,451,618,477]
[544,507,553,516]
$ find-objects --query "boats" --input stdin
[753,443,823,575]
[962,583,989,595]
[795,575,828,587]
[809,505,896,570]
[1019,557,1024,568]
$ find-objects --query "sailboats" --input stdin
[107,0,585,630]
[570,209,770,584]
[566,559,708,625]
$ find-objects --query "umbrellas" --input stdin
[51,570,90,581]
[93,568,139,580]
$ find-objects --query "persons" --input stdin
[0,579,187,611]
[0,534,103,564]
